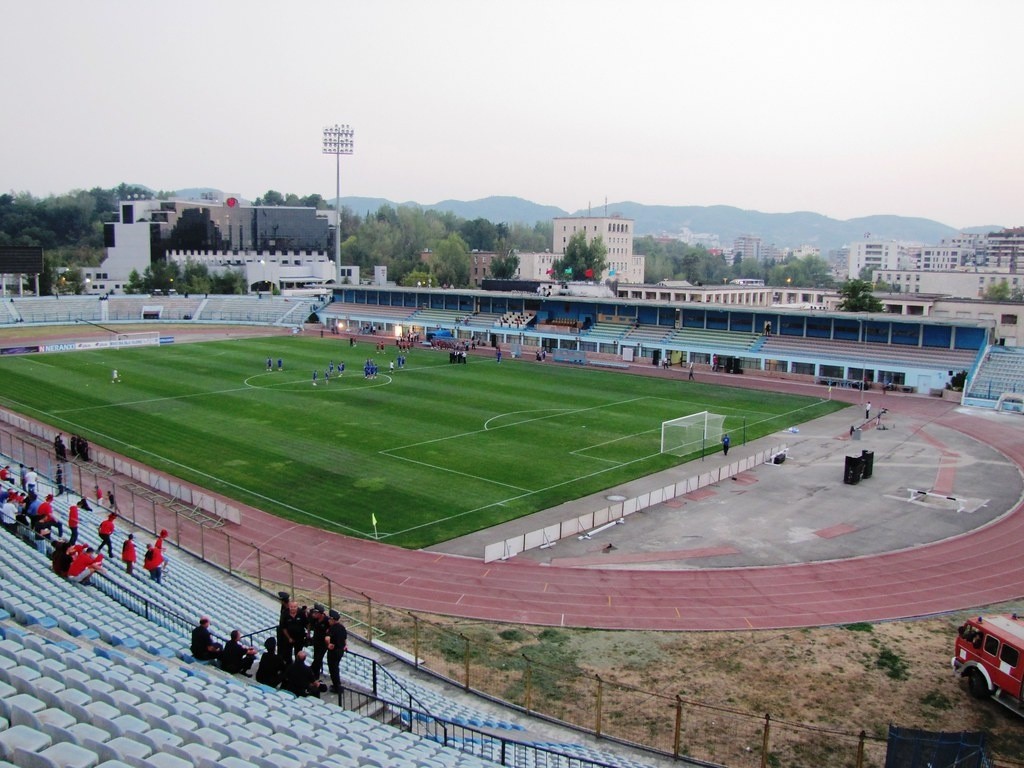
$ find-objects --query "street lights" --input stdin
[2,273,6,298]
[35,272,40,297]
[322,123,354,286]
[19,273,23,298]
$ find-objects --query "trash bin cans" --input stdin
[844,450,875,485]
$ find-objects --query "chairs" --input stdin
[0,458,674,768]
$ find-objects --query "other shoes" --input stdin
[242,673,252,678]
[162,568,168,572]
[83,582,91,587]
[330,685,344,694]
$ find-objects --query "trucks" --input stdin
[951,613,1024,718]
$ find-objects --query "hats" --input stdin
[312,604,325,614]
[45,494,54,502]
[160,530,168,538]
[277,592,290,602]
[108,512,117,521]
[329,609,341,621]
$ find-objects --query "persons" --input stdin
[267,357,273,372]
[256,591,348,698]
[713,355,718,371]
[112,368,122,383]
[722,434,729,455]
[313,311,548,386]
[635,319,640,327]
[765,324,771,336]
[45,485,169,584]
[865,401,871,419]
[53,432,89,463]
[278,358,283,371]
[688,362,694,380]
[221,630,257,678]
[0,462,63,540]
[190,617,224,660]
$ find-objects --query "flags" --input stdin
[585,270,592,277]
[565,268,572,274]
[609,270,615,276]
[546,269,555,275]
